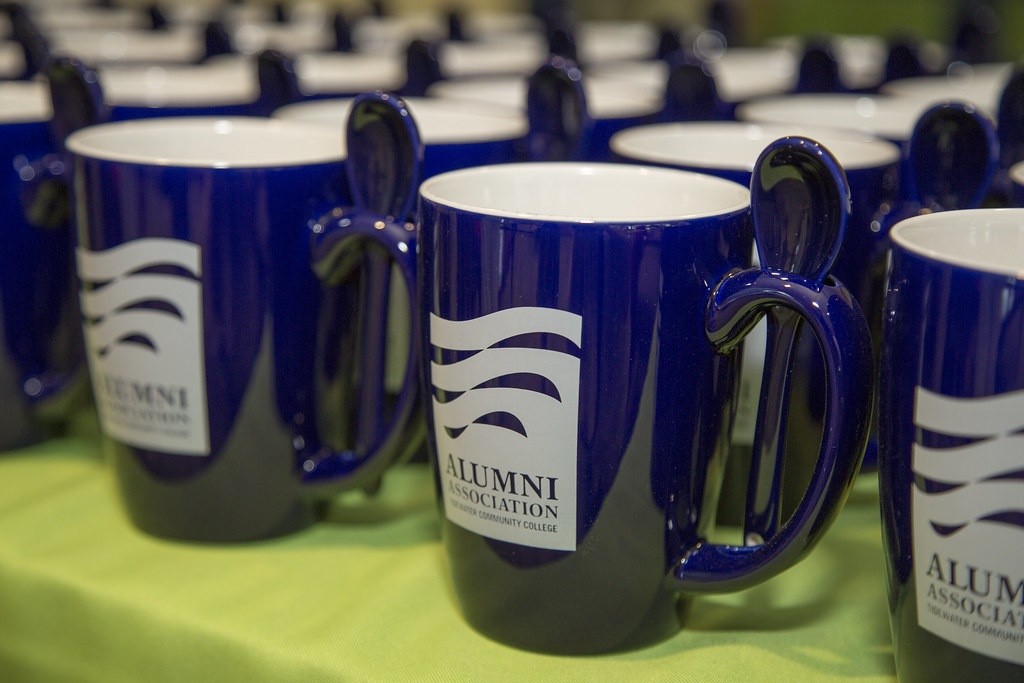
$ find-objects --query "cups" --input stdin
[0,0,1024,683]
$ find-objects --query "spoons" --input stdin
[893,99,1004,215]
[522,59,593,161]
[665,60,716,122]
[741,134,852,550]
[44,56,107,144]
[343,89,427,499]
